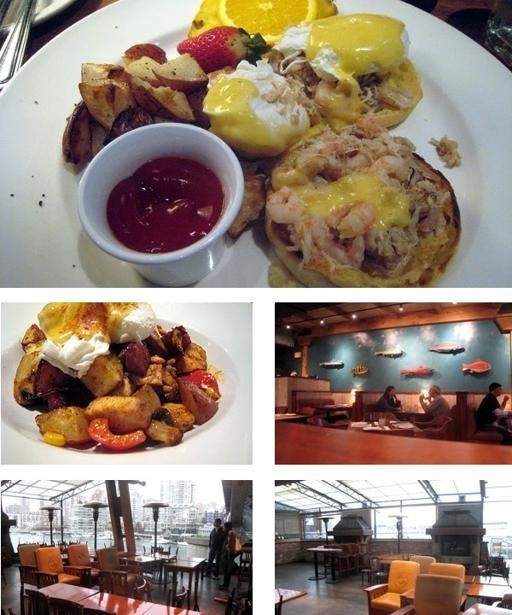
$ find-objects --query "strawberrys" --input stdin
[176,25,269,75]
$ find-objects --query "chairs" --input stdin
[324,543,512,615]
[17,541,252,615]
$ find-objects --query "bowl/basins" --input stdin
[77,121,245,287]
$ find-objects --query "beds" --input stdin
[275,588,308,615]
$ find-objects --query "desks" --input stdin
[275,421,512,464]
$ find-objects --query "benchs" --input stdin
[275,391,504,443]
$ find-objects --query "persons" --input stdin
[375,386,403,414]
[203,518,225,580]
[475,382,511,445]
[419,385,449,419]
[218,522,242,591]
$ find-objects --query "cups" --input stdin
[379,418,386,427]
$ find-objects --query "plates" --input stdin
[0,0,512,287]
[1,319,237,453]
